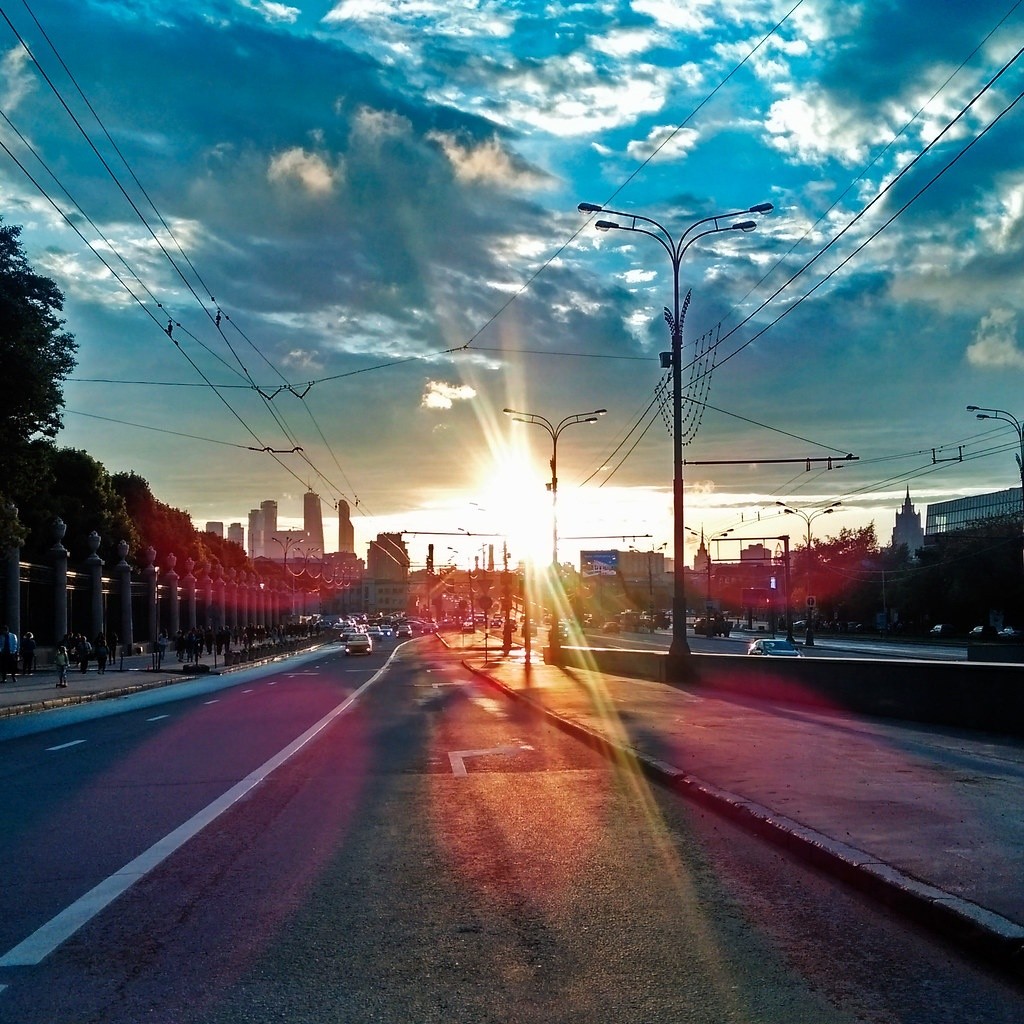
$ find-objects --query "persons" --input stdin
[94,629,118,675]
[215,626,234,655]
[715,613,723,637]
[0,625,18,682]
[234,623,289,647]
[289,623,320,638]
[174,625,215,663]
[54,646,69,686]
[20,632,35,675]
[159,631,168,660]
[63,632,92,674]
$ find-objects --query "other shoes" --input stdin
[11,675,17,682]
[2,677,7,683]
[64,682,68,687]
[59,682,64,687]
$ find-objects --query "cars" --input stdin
[584,618,599,627]
[602,621,620,634]
[792,619,901,634]
[970,627,985,634]
[998,627,1016,635]
[931,625,948,633]
[296,610,570,655]
[747,637,806,658]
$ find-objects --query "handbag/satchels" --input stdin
[87,644,95,662]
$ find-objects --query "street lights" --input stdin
[576,200,777,686]
[685,526,733,637]
[628,542,669,634]
[584,555,618,629]
[271,537,359,616]
[447,502,510,638]
[502,408,607,663]
[777,501,843,644]
[966,406,1024,493]
